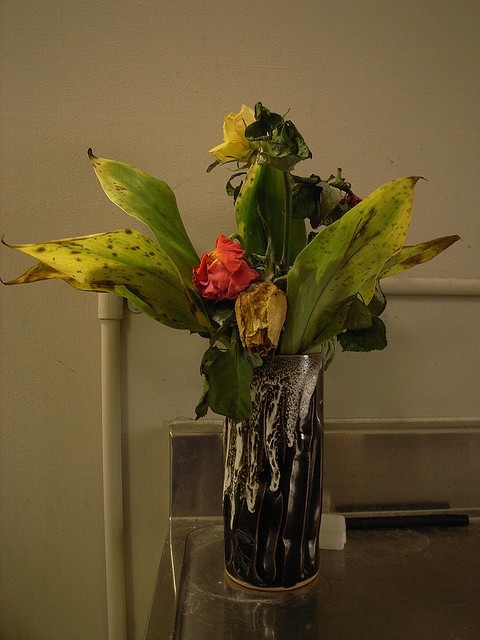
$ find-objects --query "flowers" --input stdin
[3,101,460,590]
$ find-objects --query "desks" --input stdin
[167,419,480,638]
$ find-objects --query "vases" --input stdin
[223,353,325,587]
